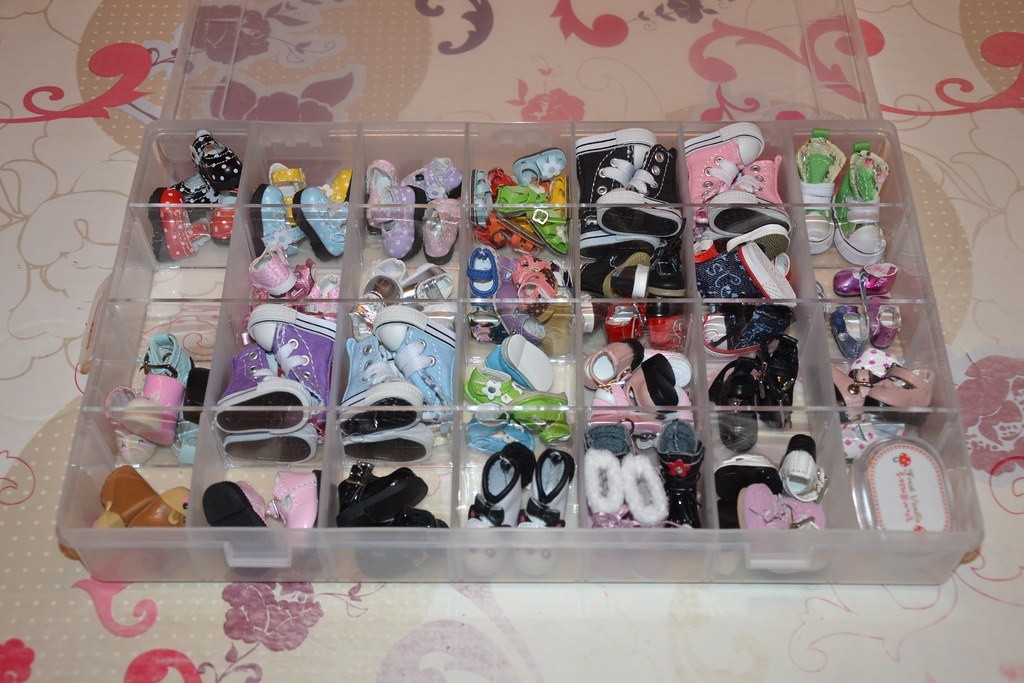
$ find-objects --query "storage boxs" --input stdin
[54,1,986,587]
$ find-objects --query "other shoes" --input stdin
[87,124,959,530]
